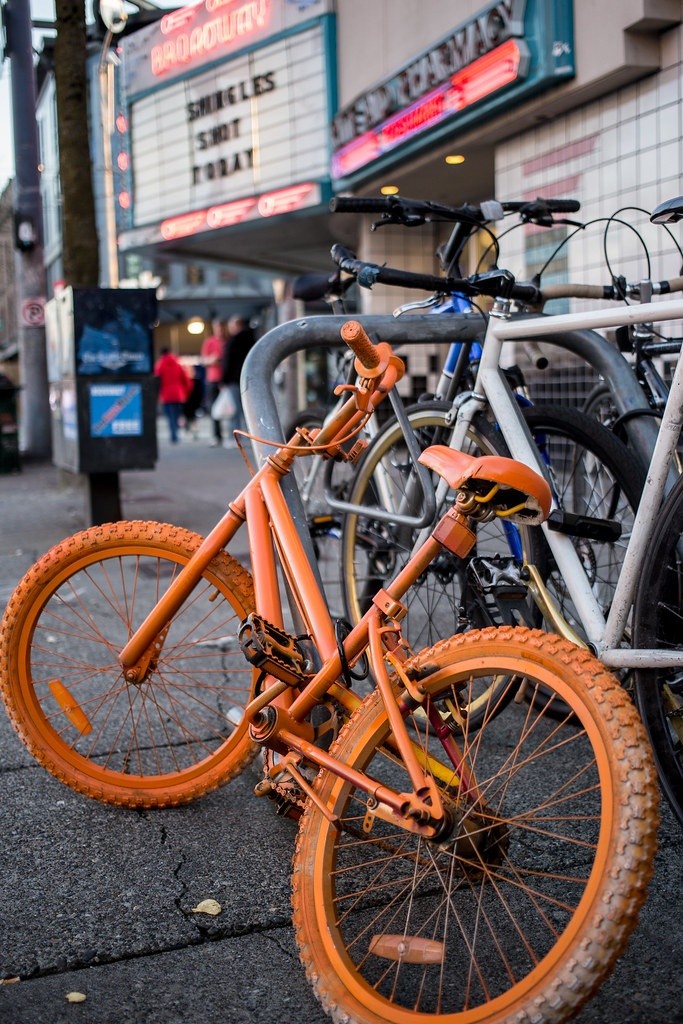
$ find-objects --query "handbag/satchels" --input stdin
[211,388,237,420]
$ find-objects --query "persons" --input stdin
[154,314,257,448]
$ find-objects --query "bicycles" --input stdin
[531,195,683,615]
[2,319,661,1024]
[328,178,659,724]
[321,236,682,837]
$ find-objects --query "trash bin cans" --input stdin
[42,282,159,473]
